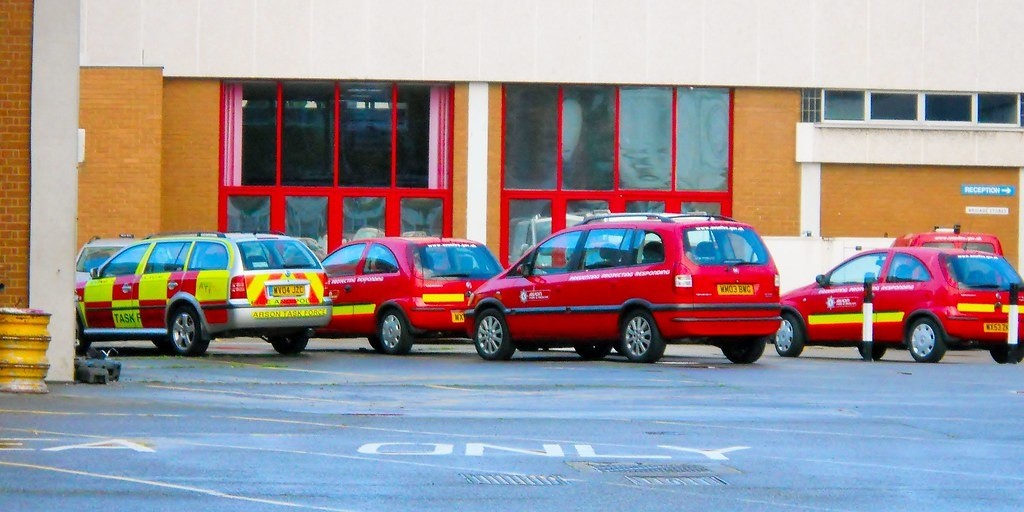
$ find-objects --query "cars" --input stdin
[305,237,505,355]
[520,213,584,268]
[565,208,719,266]
[767,247,1024,365]
[876,225,1003,281]
[269,237,326,268]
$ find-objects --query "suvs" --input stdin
[75,231,333,356]
[75,234,152,284]
[463,212,783,364]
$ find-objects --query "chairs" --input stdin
[895,265,912,281]
[599,243,618,268]
[415,254,434,268]
[696,241,716,265]
[248,255,269,268]
[641,241,664,264]
[375,255,394,272]
